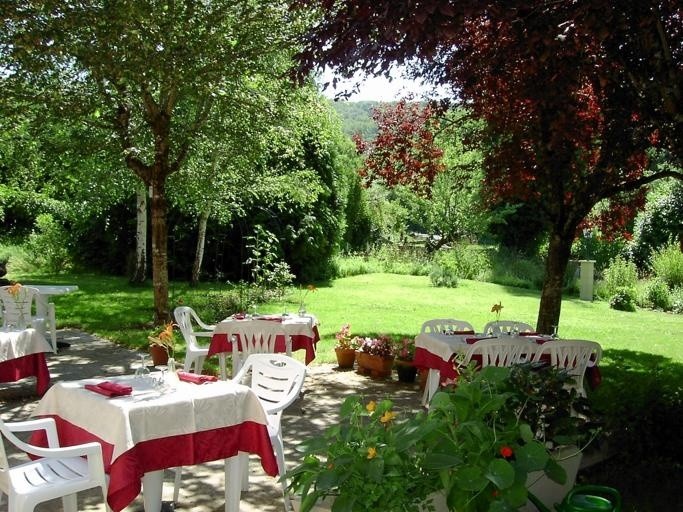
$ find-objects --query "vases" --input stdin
[369,355,394,380]
[357,352,373,376]
[396,361,416,383]
[335,347,355,368]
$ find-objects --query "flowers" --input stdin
[396,338,414,361]
[356,337,375,352]
[334,322,357,349]
[375,336,397,355]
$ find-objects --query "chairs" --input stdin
[483,319,536,332]
[456,337,532,367]
[533,339,603,422]
[420,319,473,333]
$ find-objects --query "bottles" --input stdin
[299,300,306,318]
[17,312,26,331]
[167,357,179,392]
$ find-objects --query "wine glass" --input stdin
[154,365,170,393]
[134,353,152,390]
[282,306,288,316]
[252,306,260,316]
[511,321,519,339]
[551,325,559,341]
